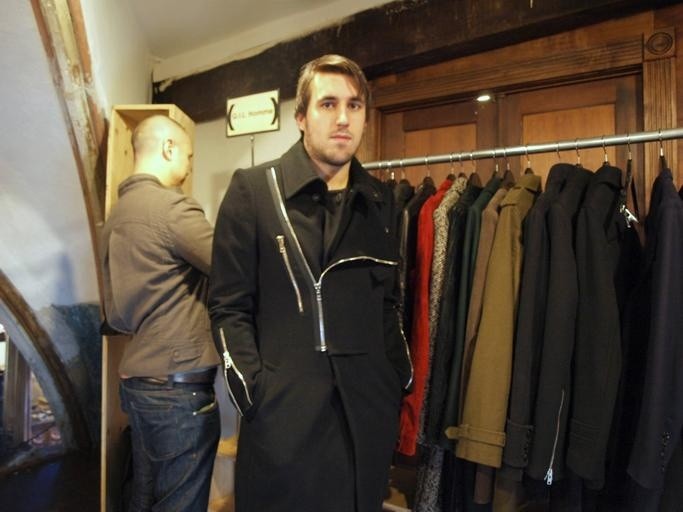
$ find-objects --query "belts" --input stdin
[154,368,216,384]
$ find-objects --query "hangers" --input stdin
[377,128,667,222]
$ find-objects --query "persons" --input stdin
[101,114,219,512]
[205,53,416,511]
